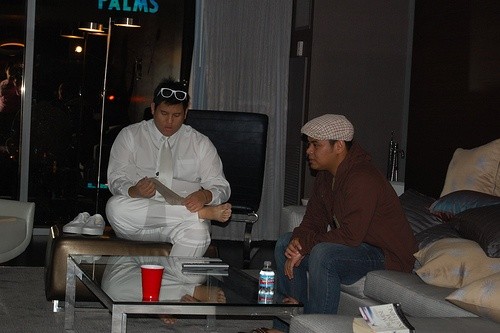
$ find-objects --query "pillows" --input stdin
[397,137,500,322]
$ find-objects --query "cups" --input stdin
[140,264,164,302]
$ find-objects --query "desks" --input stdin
[65,253,305,333]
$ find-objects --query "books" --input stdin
[352,302,416,333]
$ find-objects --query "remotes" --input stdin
[182,262,229,267]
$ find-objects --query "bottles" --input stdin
[257,290,274,304]
[260,261,275,291]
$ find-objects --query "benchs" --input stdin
[45,224,218,312]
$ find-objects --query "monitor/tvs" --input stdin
[186,109,269,214]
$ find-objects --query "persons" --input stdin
[273,116,417,317]
[106,77,232,322]
[103,258,228,303]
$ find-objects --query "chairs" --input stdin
[184,109,268,261]
[0,198,36,262]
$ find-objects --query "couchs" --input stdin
[280,205,500,333]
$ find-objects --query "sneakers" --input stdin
[81,214,106,236]
[63,212,91,234]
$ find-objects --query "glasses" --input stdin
[156,87,188,102]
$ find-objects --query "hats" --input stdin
[300,113,355,142]
